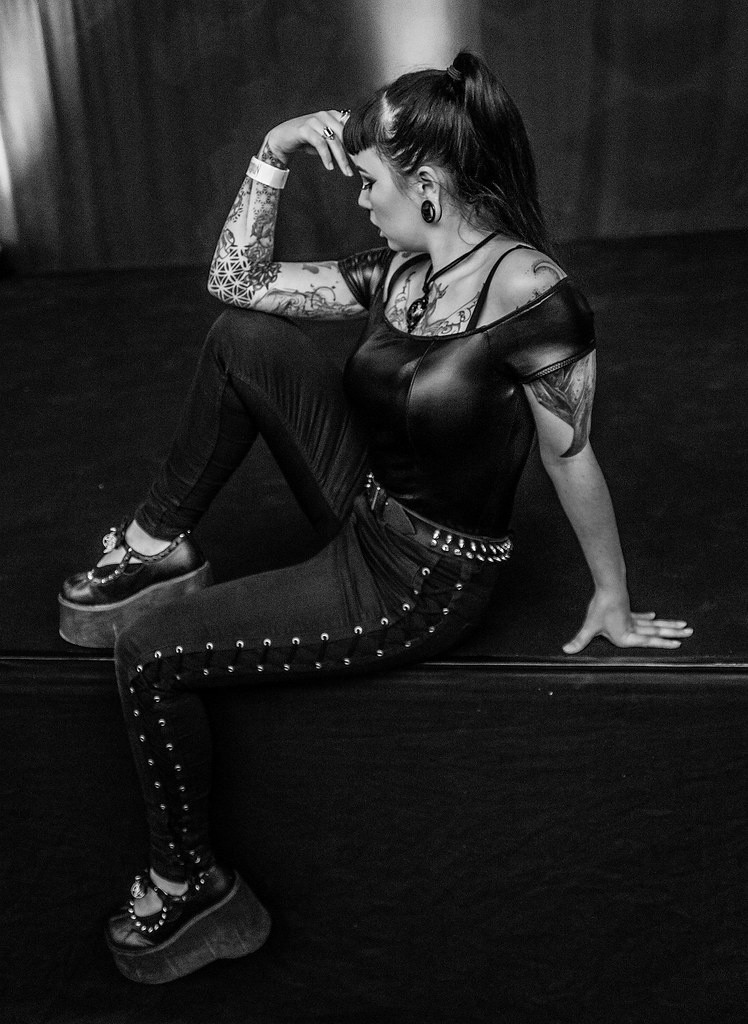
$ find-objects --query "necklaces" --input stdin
[407,229,502,334]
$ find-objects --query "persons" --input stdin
[56,49,695,985]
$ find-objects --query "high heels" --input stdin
[103,862,269,987]
[55,520,213,651]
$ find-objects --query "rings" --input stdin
[338,108,351,122]
[322,127,335,140]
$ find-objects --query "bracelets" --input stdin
[245,155,290,190]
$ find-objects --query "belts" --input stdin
[353,474,514,569]
